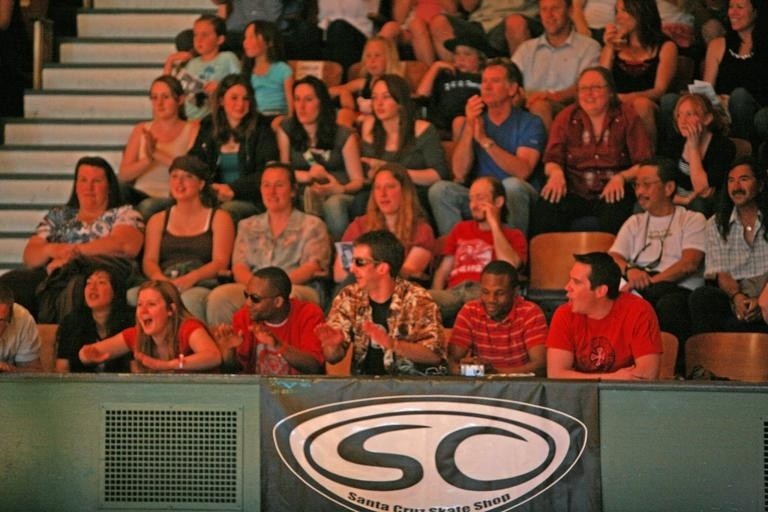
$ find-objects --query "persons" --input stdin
[2,0,768,384]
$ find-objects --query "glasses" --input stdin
[625,238,663,270]
[352,255,377,267]
[242,288,278,305]
[576,83,608,94]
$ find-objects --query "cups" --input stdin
[612,22,628,50]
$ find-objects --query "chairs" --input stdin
[37,61,767,382]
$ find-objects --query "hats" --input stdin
[168,156,210,181]
[443,20,502,59]
